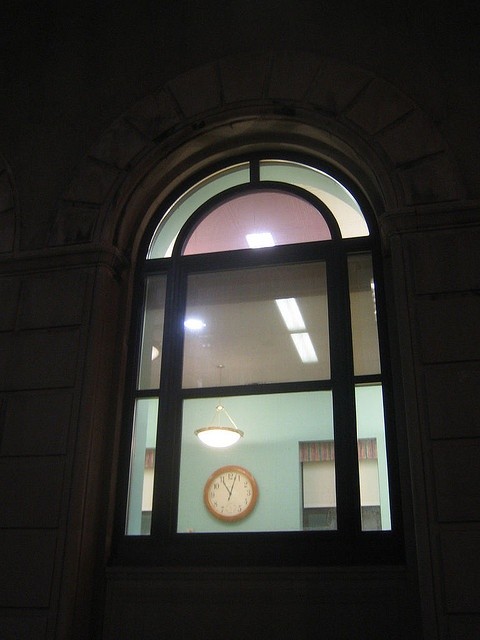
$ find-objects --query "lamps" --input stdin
[194,366,245,448]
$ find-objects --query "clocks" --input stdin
[203,465,259,521]
